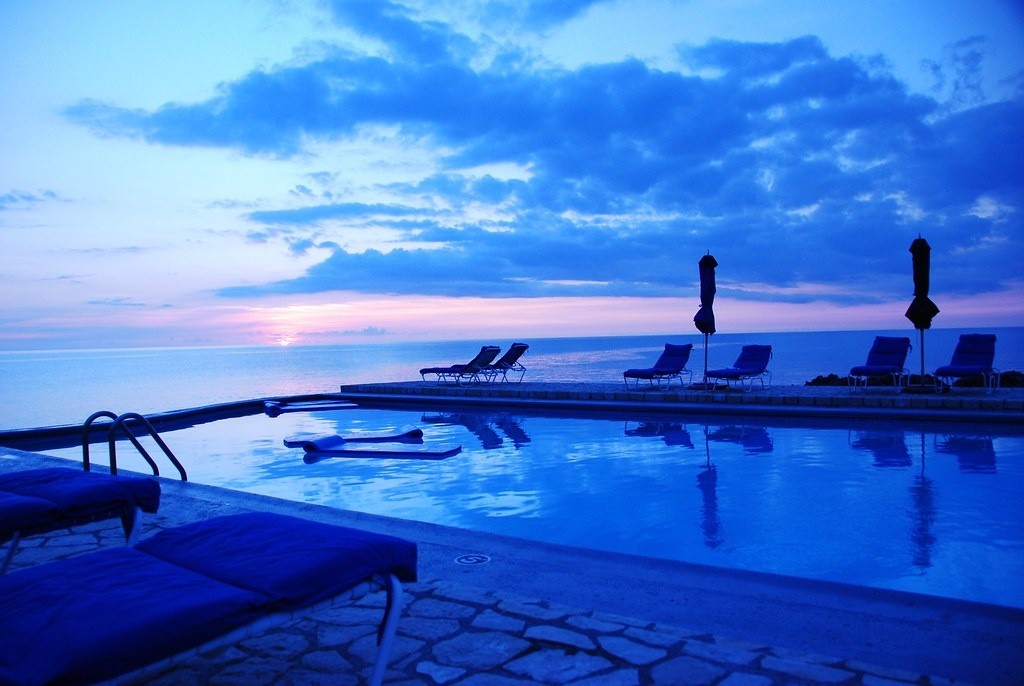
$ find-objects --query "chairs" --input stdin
[624,343,694,391]
[848,428,913,468]
[849,336,912,394]
[623,419,695,450]
[934,432,998,476]
[420,346,501,387]
[933,334,999,394]
[0,512,418,686]
[422,407,533,449]
[452,343,529,384]
[704,345,773,393]
[0,467,161,576]
[709,421,774,456]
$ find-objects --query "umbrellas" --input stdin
[904,233,941,386]
[693,249,719,384]
[695,424,722,549]
[909,433,938,578]
